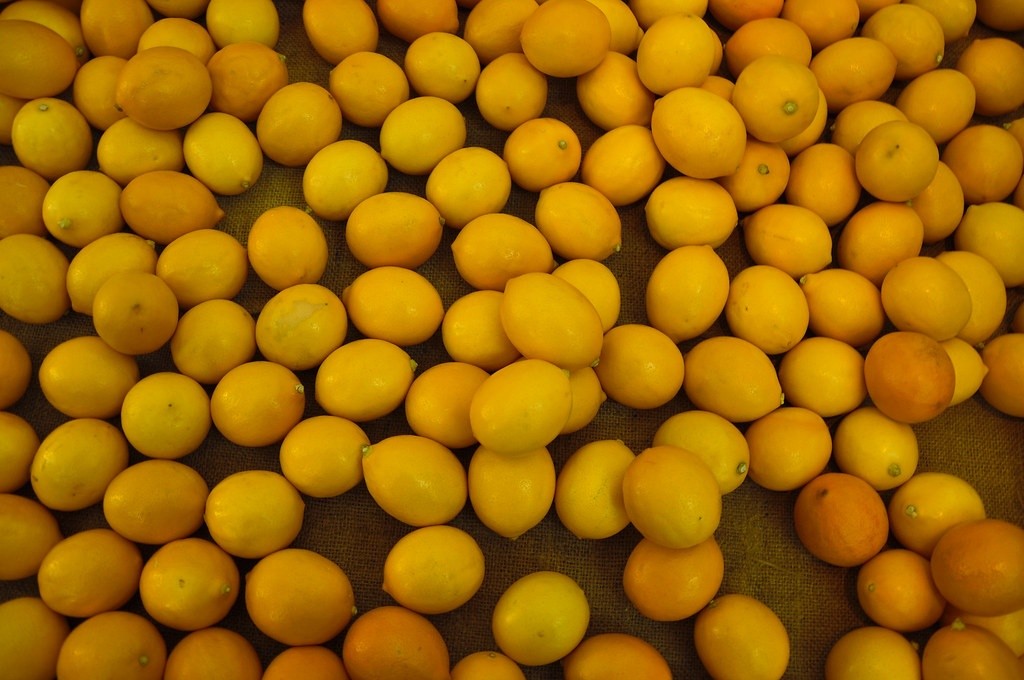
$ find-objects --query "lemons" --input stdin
[0,0,1024,680]
